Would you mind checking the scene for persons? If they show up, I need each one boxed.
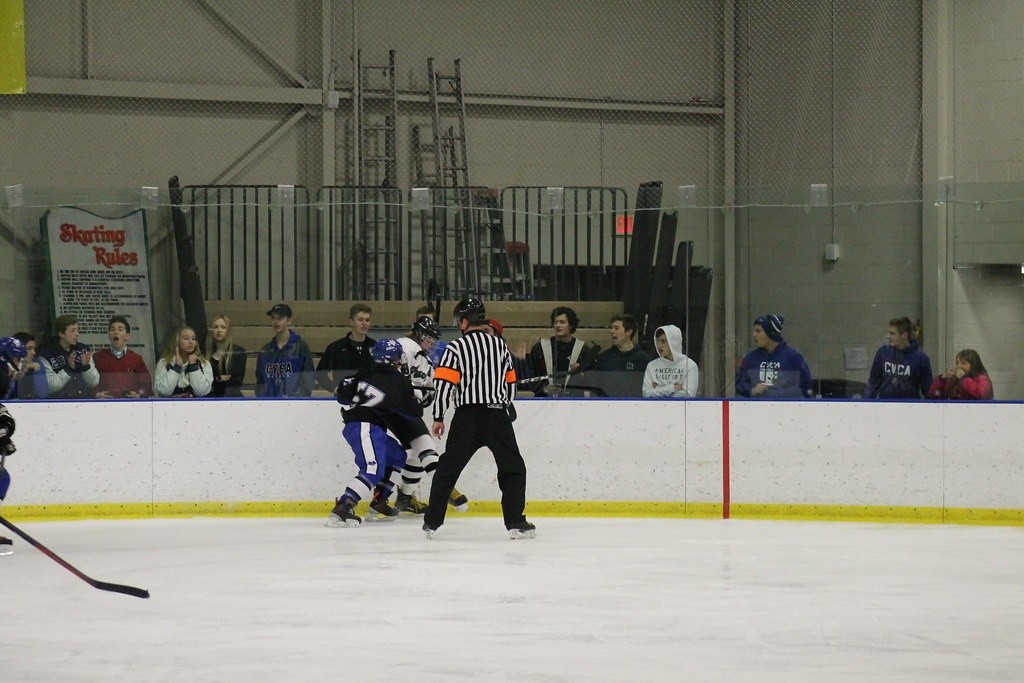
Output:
[204,311,247,398]
[483,319,534,391]
[255,303,315,398]
[734,315,815,399]
[865,314,934,400]
[640,325,699,398]
[321,316,468,528]
[416,307,447,372]
[316,303,377,397]
[0,337,28,558]
[153,324,213,397]
[928,349,995,400]
[525,303,595,398]
[87,317,151,399]
[596,314,651,373]
[422,297,536,540]
[8,332,48,399]
[38,313,101,399]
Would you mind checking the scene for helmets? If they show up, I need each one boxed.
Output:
[410,316,441,355]
[374,339,403,364]
[454,298,486,324]
[0,336,27,364]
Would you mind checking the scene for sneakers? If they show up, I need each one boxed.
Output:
[424,521,442,539]
[448,488,468,511]
[0,536,13,555]
[324,497,362,527]
[365,488,399,521]
[395,485,428,516]
[508,522,536,538]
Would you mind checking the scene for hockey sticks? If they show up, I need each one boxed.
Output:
[0,515,151,599]
[516,345,601,385]
[435,292,441,323]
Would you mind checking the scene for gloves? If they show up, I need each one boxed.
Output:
[0,429,15,455]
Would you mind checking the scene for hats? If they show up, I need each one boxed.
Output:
[488,319,503,335]
[759,315,784,340]
[267,304,292,318]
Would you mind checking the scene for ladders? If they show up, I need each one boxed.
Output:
[412,124,535,300]
[341,48,402,301]
[427,56,478,300]
